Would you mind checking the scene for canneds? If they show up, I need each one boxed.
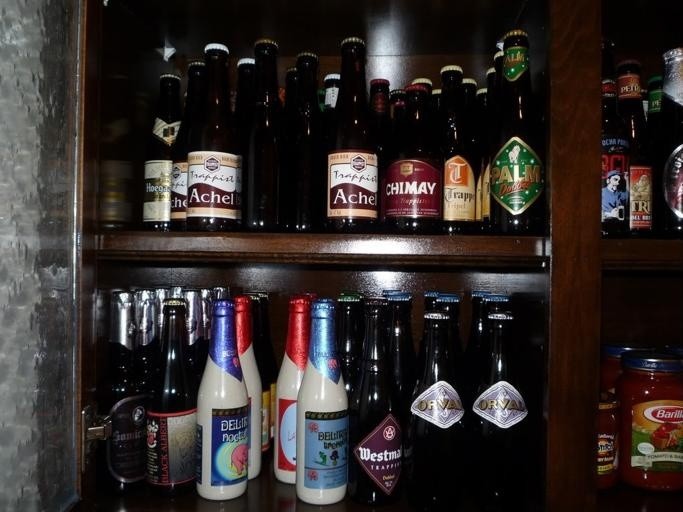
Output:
[596,341,683,500]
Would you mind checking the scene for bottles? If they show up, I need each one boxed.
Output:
[98,278,530,511]
[85,288,105,373]
[601,45,681,236]
[138,29,546,233]
[99,67,140,239]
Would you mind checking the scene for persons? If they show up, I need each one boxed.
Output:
[601,169,633,226]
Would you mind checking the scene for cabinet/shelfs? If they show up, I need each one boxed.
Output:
[577,0,682,512]
[72,0,577,511]
